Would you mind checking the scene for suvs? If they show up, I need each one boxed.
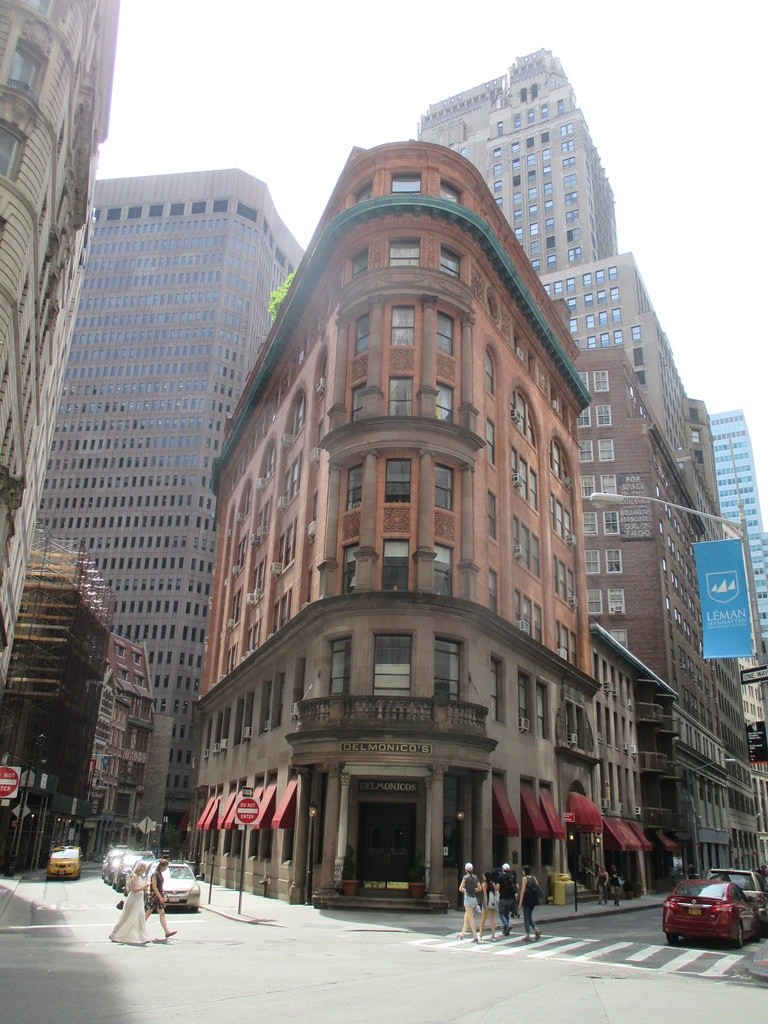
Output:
[706,868,768,938]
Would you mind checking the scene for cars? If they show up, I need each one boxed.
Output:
[662,879,762,949]
[144,862,203,913]
[101,844,198,897]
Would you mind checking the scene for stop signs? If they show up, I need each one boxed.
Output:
[0,767,19,798]
[235,797,260,823]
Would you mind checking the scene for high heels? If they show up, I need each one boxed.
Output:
[457,934,464,940]
[471,937,477,943]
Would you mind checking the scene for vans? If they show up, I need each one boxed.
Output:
[47,845,84,881]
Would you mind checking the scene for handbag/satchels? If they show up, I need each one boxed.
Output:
[161,892,169,902]
[487,890,497,909]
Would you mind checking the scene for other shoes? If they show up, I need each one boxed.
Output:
[598,900,619,906]
[522,937,532,942]
[535,929,541,940]
[511,913,520,918]
[504,925,512,936]
[165,931,177,938]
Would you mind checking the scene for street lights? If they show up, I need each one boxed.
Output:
[590,491,768,742]
[689,758,737,879]
[5,744,50,878]
[79,768,100,845]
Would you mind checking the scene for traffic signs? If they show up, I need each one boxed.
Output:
[242,786,254,798]
[740,663,768,685]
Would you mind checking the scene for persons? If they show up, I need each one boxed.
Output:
[145,859,178,939]
[494,863,519,936]
[518,865,545,941]
[594,865,608,905]
[611,864,626,906]
[108,862,151,944]
[761,862,767,880]
[457,862,483,943]
[476,871,500,941]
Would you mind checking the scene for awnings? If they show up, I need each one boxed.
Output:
[653,833,678,851]
[568,791,603,832]
[519,781,564,840]
[177,808,189,832]
[270,772,298,830]
[604,818,652,852]
[491,775,518,837]
[251,778,277,830]
[216,784,246,829]
[197,790,224,831]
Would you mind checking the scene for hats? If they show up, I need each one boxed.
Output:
[465,863,473,871]
[502,863,510,871]
